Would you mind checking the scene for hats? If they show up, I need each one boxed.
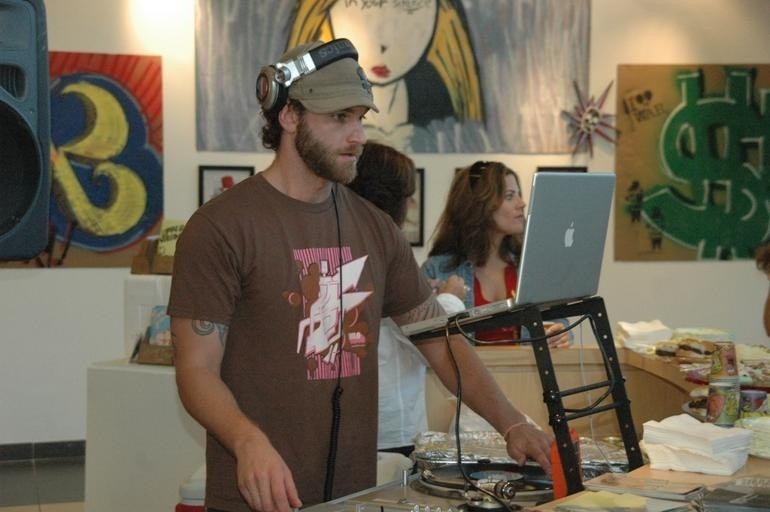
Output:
[277,41,379,115]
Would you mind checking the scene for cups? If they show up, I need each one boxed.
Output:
[741,390,767,411]
[709,341,740,396]
[705,382,739,426]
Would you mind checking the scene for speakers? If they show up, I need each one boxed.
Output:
[0,0,51,262]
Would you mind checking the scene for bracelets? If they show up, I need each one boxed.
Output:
[503,420,538,442]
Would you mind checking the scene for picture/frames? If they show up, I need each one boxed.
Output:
[195,163,257,206]
[404,168,427,248]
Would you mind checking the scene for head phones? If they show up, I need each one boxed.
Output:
[255,38,358,113]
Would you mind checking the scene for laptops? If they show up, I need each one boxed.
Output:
[401,172,616,337]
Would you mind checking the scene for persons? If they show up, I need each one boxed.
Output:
[161,36,557,511]
[333,138,432,468]
[417,160,577,355]
[271,1,489,135]
